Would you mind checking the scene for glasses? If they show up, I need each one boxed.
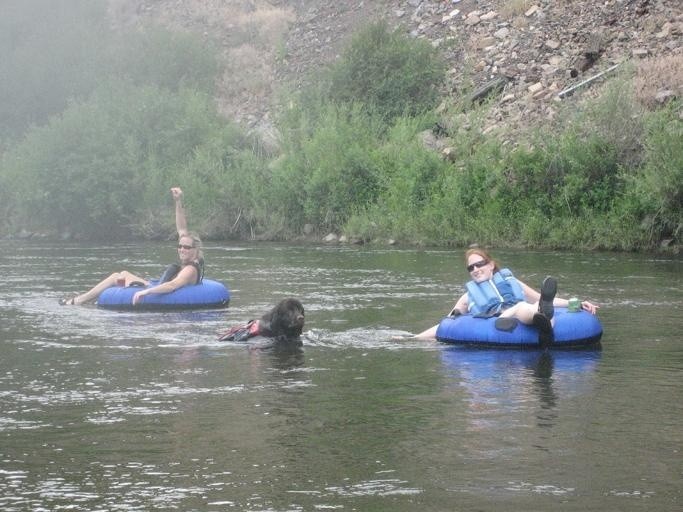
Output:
[466,259,490,273]
[177,243,197,251]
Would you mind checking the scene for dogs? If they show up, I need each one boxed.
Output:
[216,298,306,342]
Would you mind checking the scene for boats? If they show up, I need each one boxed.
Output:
[435,305,604,351]
[97,277,232,311]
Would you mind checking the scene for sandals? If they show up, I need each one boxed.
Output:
[58,298,76,306]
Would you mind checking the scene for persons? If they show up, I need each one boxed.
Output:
[418,248,600,346]
[59,187,205,306]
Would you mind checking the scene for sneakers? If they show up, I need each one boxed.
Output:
[537,276,557,320]
[532,313,555,347]
